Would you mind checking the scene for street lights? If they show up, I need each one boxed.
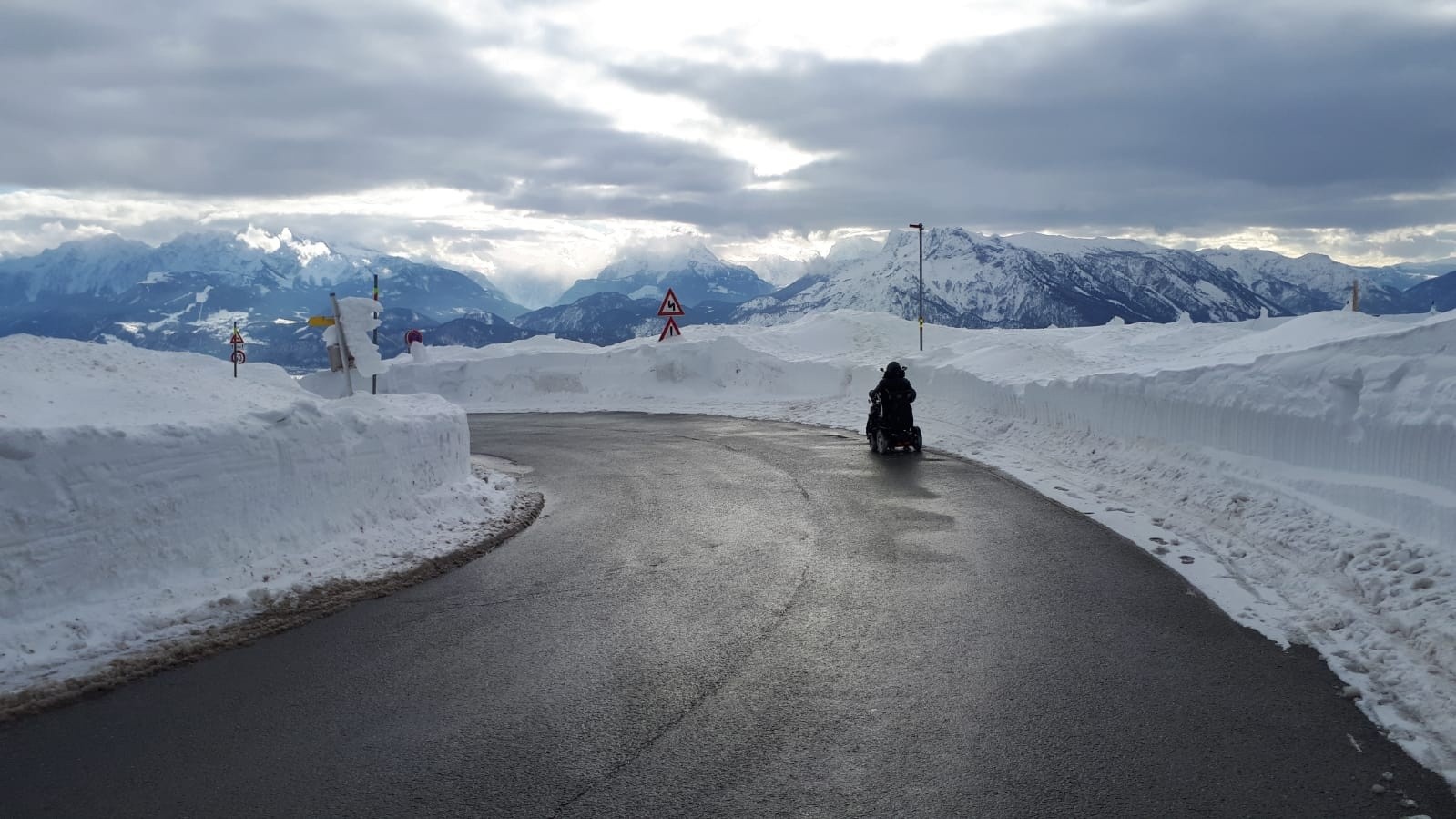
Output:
[910,223,926,350]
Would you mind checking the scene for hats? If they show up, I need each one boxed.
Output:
[887,362,900,372]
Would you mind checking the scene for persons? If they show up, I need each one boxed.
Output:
[869,363,915,432]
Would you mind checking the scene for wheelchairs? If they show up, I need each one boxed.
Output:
[865,389,924,454]
